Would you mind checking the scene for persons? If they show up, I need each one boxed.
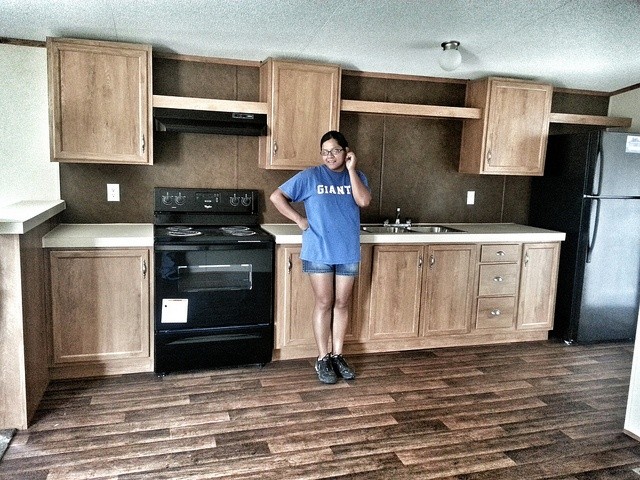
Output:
[269,130,373,383]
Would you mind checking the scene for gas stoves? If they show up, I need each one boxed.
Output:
[155,224,276,244]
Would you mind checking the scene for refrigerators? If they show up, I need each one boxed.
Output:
[527,132,639,344]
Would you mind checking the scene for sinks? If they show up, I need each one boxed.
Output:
[408,223,467,232]
[363,224,411,234]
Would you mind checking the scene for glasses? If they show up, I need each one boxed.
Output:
[320,148,345,155]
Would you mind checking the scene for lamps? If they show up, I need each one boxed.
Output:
[438,41,462,71]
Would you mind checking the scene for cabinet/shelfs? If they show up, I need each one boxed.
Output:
[278,245,362,346]
[457,75,554,177]
[42,246,154,382]
[515,242,560,330]
[257,56,343,171]
[367,243,477,341]
[472,243,521,331]
[45,35,156,166]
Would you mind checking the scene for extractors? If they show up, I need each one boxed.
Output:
[155,108,268,137]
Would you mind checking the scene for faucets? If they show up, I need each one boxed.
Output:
[396,207,403,225]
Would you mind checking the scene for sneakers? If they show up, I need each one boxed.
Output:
[332,354,356,379]
[315,353,337,383]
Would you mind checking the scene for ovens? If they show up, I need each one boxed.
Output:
[152,245,273,377]
